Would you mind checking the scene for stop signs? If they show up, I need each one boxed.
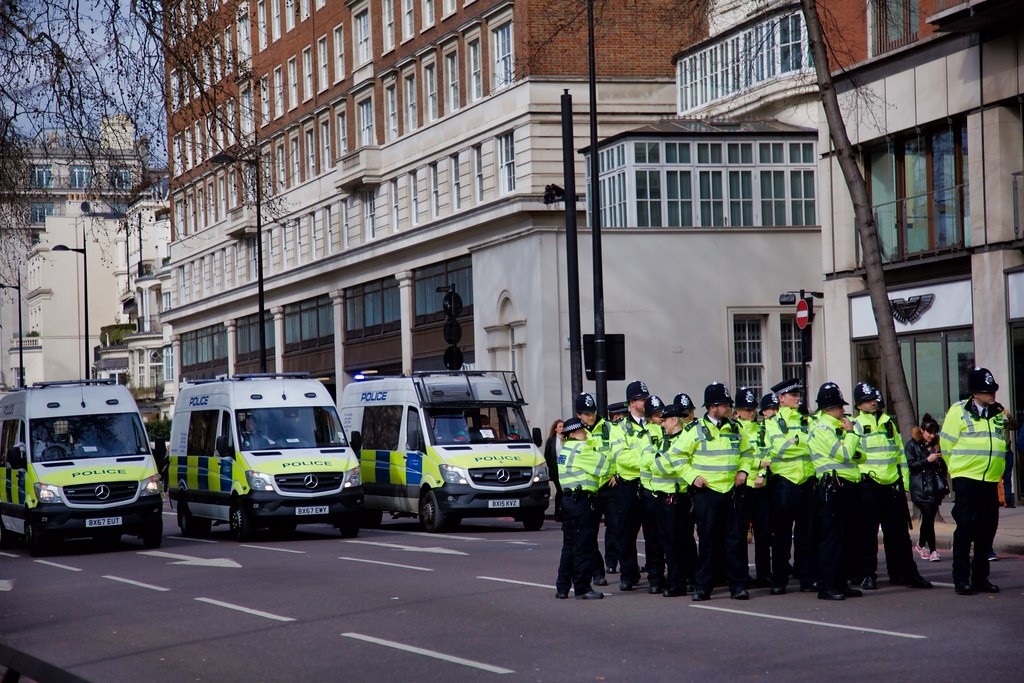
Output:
[796,299,809,329]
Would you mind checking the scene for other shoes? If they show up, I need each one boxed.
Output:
[554,514,560,522]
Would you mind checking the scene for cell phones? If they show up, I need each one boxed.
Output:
[936,451,941,455]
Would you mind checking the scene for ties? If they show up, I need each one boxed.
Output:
[982,409,986,417]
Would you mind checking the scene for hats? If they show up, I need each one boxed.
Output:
[561,418,588,436]
[771,378,804,398]
[606,402,629,414]
[660,405,685,419]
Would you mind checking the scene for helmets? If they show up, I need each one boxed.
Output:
[759,393,780,416]
[627,381,650,402]
[734,388,758,410]
[575,392,597,413]
[701,382,734,408]
[816,382,850,412]
[644,395,665,417]
[854,384,877,410]
[967,368,999,393]
[674,393,696,414]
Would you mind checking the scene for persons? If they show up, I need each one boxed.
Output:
[241,415,275,444]
[939,368,1006,594]
[1003,409,1018,508]
[555,378,932,600]
[473,417,498,438]
[905,412,946,560]
[544,419,565,521]
[162,455,169,495]
[13,425,48,462]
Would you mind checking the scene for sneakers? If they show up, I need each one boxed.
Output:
[930,551,940,562]
[915,541,929,559]
[988,551,998,560]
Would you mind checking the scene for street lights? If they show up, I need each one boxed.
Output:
[52,224,90,386]
[211,126,267,373]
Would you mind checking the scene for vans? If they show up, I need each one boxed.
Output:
[338,369,551,532]
[168,372,365,538]
[0,380,164,556]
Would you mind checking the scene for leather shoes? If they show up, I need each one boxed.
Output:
[555,566,999,601]
